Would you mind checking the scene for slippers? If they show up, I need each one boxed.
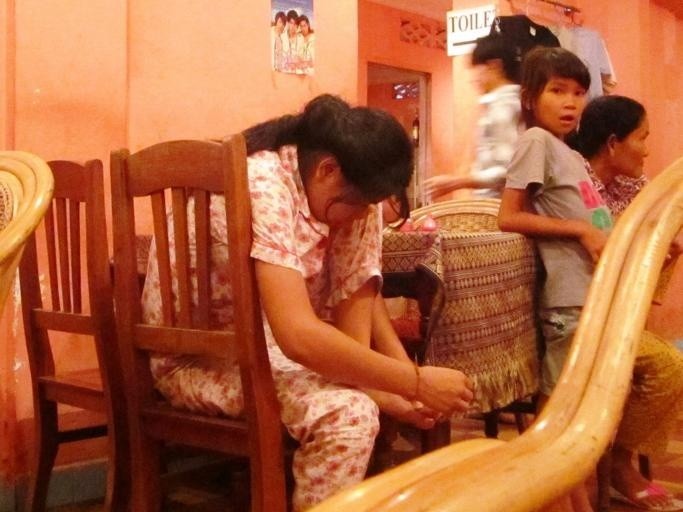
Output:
[610,483,682,511]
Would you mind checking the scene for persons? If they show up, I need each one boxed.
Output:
[422,33,523,201]
[565,94,683,510]
[272,9,314,74]
[497,46,616,512]
[401,366,419,401]
[140,94,475,512]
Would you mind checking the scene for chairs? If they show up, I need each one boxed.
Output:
[418,175,538,437]
[110,135,396,512]
[17,158,123,512]
[1,148,55,512]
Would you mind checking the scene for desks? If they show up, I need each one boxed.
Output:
[110,230,543,455]
